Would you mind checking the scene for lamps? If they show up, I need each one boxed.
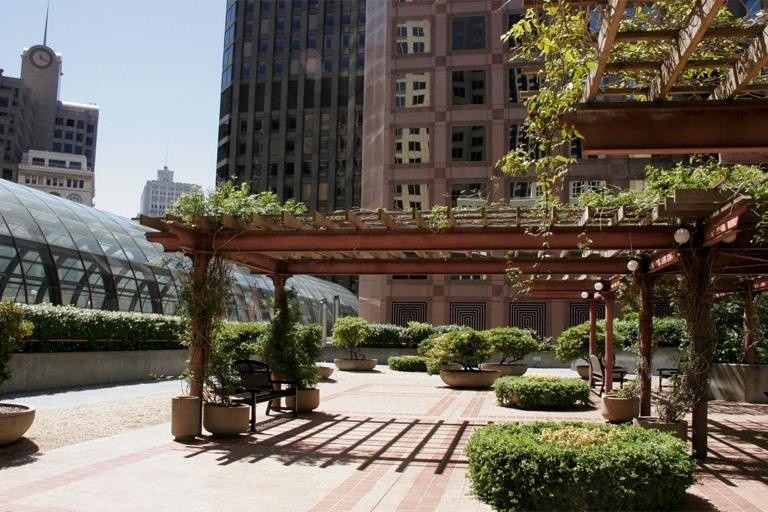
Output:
[624,256,641,273]
[579,290,590,299]
[592,280,604,292]
[672,221,691,247]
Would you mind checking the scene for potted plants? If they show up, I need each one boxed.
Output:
[0,296,40,446]
[415,331,464,373]
[427,330,503,390]
[277,322,321,415]
[329,314,379,372]
[488,372,596,411]
[479,323,554,378]
[295,320,332,380]
[182,320,256,437]
[601,371,645,422]
[554,315,630,379]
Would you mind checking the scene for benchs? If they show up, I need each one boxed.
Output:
[654,357,686,399]
[586,351,630,397]
[228,358,300,433]
